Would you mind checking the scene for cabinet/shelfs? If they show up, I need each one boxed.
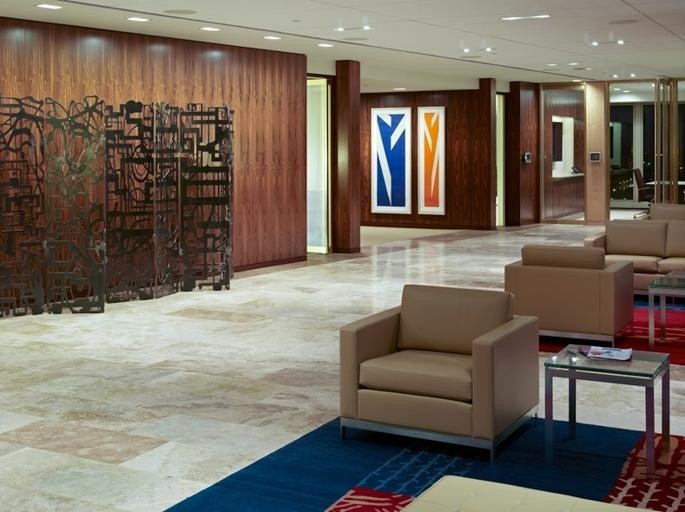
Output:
[552,180,585,218]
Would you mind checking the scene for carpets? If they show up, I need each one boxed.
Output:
[162,415,684,510]
[539,296,685,365]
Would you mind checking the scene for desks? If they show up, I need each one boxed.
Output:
[644,180,685,201]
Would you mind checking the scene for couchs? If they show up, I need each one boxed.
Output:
[584,203,685,298]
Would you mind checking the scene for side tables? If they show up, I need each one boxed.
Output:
[536,341,672,480]
[645,270,685,345]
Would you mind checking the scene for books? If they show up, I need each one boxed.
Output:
[568,349,587,360]
[587,345,633,362]
[579,346,615,361]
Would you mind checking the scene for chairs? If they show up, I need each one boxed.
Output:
[633,169,656,219]
[505,243,635,351]
[334,282,540,463]
